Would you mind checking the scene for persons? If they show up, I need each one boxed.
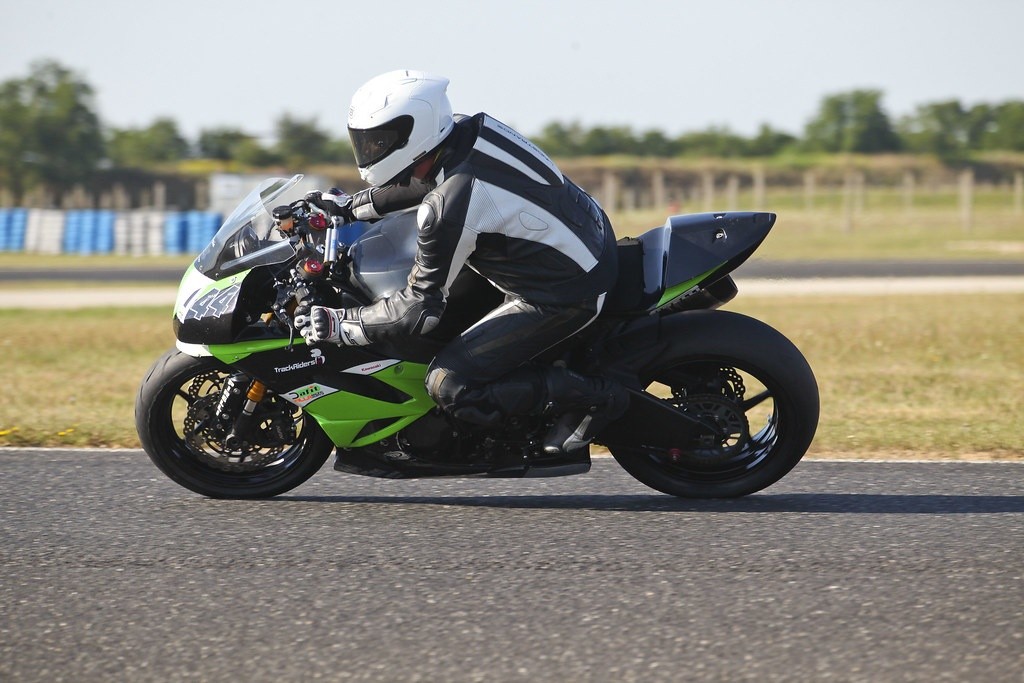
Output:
[295,65,630,452]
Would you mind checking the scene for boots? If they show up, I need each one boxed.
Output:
[542,360,630,453]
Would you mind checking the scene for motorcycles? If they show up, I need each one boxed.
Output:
[135,174,820,501]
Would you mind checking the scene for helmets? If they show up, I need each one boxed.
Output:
[347,68,455,188]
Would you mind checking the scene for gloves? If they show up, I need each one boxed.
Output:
[293,306,373,349]
[321,186,384,227]
